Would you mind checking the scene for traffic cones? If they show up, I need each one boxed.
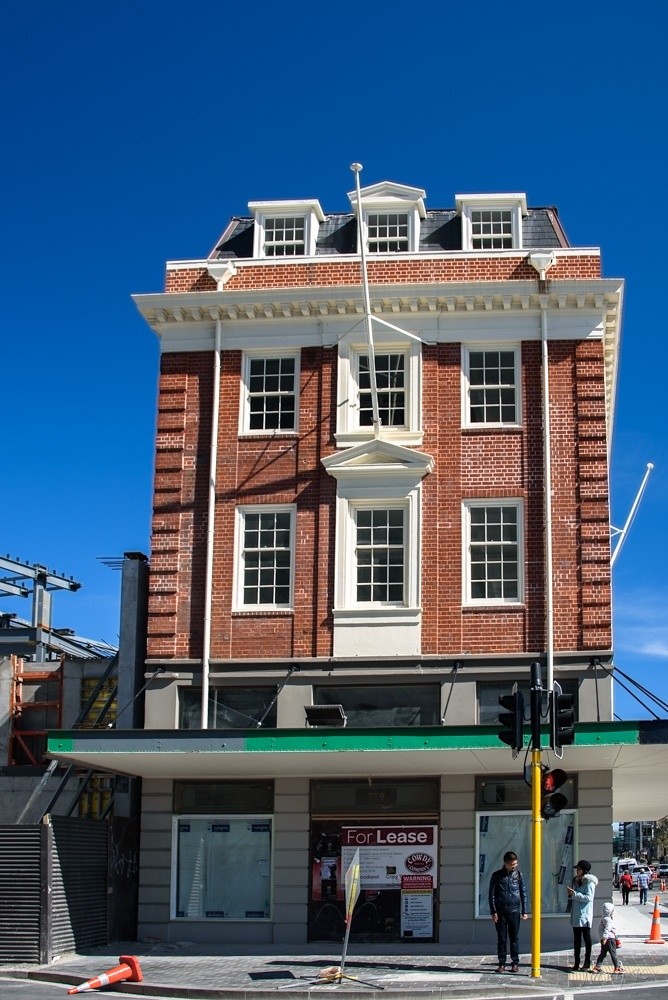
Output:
[645,896,665,944]
[653,871,657,880]
[68,954,143,995]
[663,881,666,891]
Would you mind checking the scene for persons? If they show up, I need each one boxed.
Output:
[593,902,626,973]
[619,870,632,905]
[636,868,649,905]
[566,860,599,972]
[489,851,528,972]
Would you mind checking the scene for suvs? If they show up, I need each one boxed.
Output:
[614,858,668,888]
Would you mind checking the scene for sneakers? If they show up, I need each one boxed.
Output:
[580,967,590,972]
[613,968,626,973]
[566,968,578,973]
[593,967,605,974]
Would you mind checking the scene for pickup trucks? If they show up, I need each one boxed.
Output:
[630,865,653,890]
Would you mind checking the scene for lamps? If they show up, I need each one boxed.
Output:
[304,705,347,730]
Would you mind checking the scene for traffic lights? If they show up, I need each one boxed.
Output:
[553,680,574,759]
[497,692,523,754]
[541,768,568,820]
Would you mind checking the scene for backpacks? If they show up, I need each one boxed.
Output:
[622,876,630,890]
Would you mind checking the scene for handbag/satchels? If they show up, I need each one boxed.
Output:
[616,940,621,948]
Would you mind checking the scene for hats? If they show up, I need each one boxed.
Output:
[573,860,591,874]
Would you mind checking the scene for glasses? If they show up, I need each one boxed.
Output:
[578,867,582,870]
[505,861,518,866]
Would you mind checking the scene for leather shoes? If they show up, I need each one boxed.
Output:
[496,962,505,972]
[512,963,519,972]
